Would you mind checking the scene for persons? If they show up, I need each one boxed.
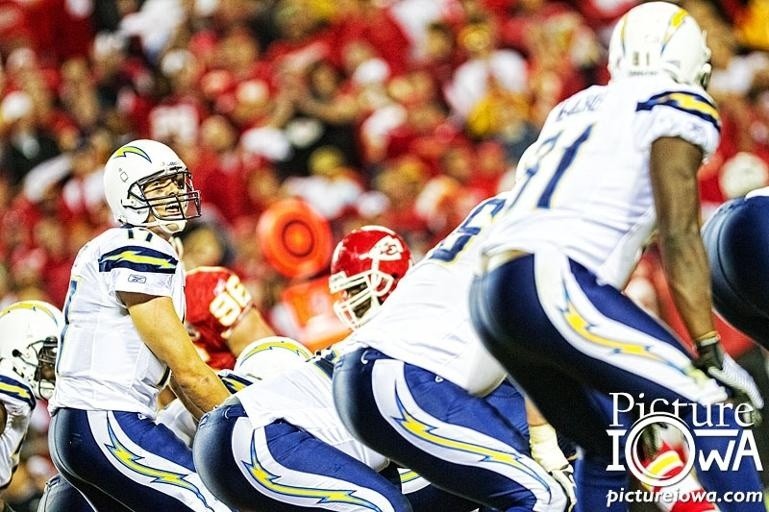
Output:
[1,1,769,511]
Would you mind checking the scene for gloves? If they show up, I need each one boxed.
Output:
[692,328,764,410]
[528,423,578,511]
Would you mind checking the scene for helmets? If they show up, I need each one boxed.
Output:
[0,300,65,402]
[607,0,712,92]
[330,223,417,332]
[102,138,201,233]
[234,335,315,381]
